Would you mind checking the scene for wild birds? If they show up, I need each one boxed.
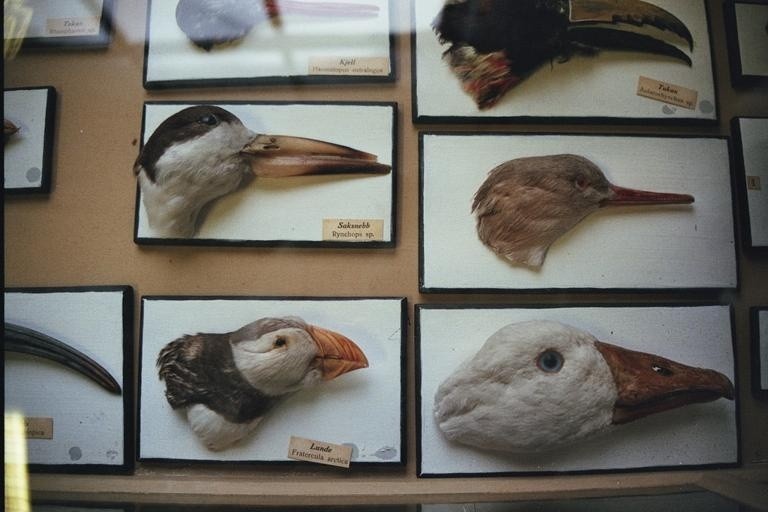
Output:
[433,319,736,455]
[176,0,380,52]
[132,105,393,238]
[471,154,695,268]
[155,317,369,453]
[431,0,694,109]
